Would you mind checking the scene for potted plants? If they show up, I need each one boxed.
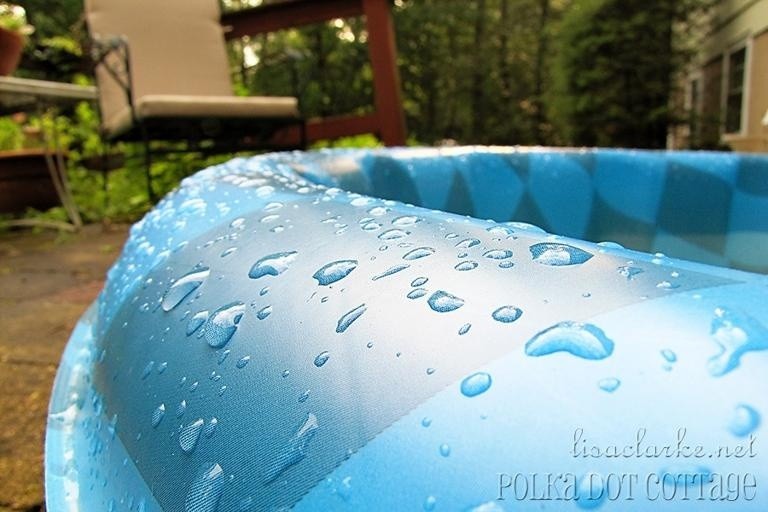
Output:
[0,2,35,77]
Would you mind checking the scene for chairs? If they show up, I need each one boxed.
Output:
[81,1,312,215]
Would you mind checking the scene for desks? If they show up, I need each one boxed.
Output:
[0,73,102,236]
